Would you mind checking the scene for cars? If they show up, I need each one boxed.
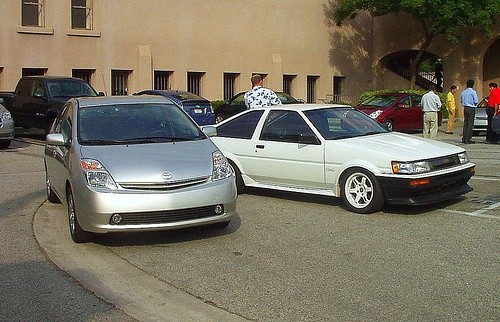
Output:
[213,91,304,124]
[42,95,237,244]
[339,93,442,133]
[133,90,216,127]
[474,96,488,135]
[199,103,476,214]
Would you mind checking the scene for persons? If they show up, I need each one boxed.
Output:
[420,86,441,140]
[480,83,500,144]
[445,85,457,134]
[461,80,479,144]
[243,74,282,109]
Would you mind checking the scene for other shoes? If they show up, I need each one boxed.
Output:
[446,132,453,134]
[485,140,489,144]
[462,139,476,143]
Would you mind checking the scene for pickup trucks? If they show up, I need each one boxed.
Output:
[0,75,106,139]
